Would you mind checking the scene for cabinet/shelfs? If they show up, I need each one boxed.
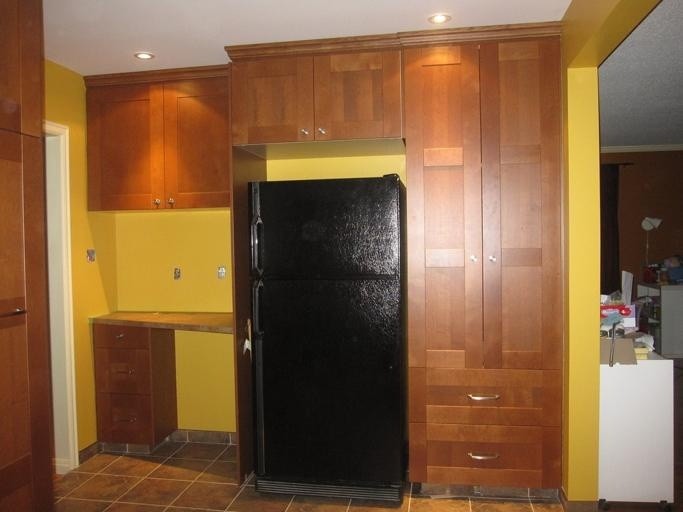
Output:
[404,424,561,487]
[230,50,405,143]
[91,328,151,450]
[83,79,235,211]
[635,283,683,359]
[407,368,561,424]
[403,38,562,370]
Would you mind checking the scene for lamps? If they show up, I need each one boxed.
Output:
[641,215,664,264]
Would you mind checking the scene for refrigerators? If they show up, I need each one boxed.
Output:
[247,177,407,501]
